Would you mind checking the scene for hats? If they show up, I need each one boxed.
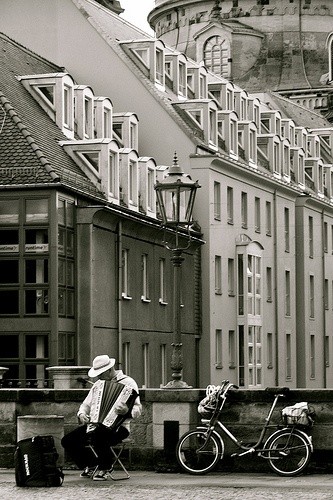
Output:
[88,355,115,378]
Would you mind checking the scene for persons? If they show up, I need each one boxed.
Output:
[62,355,142,481]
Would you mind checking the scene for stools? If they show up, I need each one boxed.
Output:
[84,444,130,481]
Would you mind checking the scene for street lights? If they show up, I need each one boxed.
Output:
[153,151,202,389]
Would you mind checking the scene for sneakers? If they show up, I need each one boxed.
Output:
[79,467,114,480]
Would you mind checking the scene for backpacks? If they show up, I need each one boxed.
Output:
[12,433,64,488]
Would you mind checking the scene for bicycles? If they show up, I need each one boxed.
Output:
[175,378,315,477]
